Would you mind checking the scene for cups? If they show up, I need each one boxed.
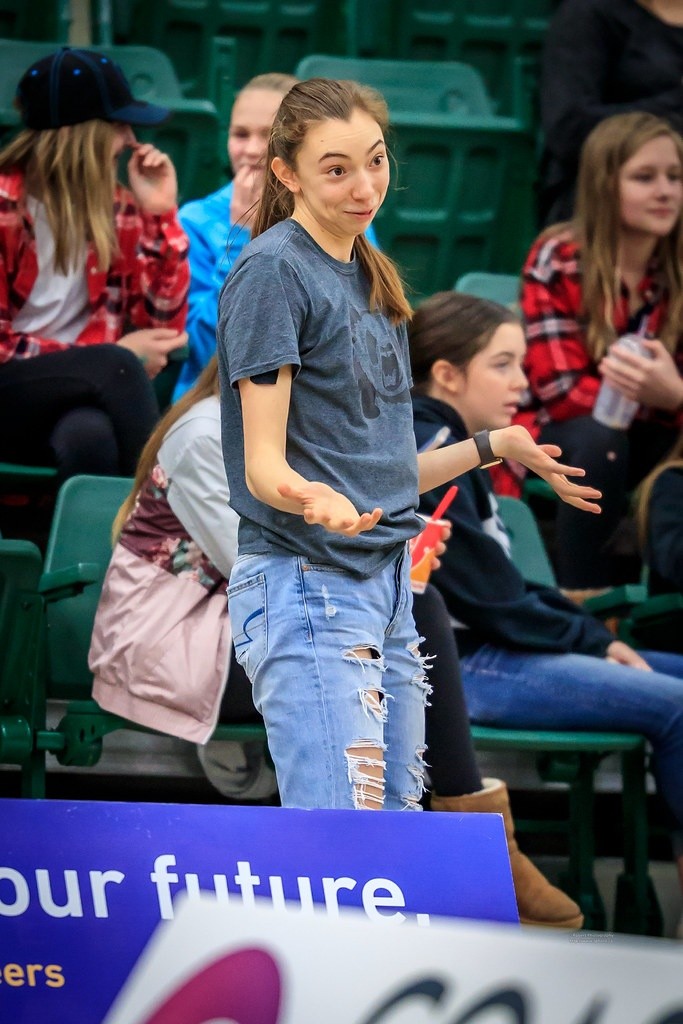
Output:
[407,512,451,595]
[592,333,658,431]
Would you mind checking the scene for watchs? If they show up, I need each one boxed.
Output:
[473,429,504,470]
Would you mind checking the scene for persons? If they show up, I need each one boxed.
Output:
[0,46,190,488]
[407,293,683,887]
[88,353,587,932]
[503,110,683,587]
[217,80,606,813]
[171,71,381,404]
[629,434,683,654]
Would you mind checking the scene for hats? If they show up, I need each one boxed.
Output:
[17,45,168,125]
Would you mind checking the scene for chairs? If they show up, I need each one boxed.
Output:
[0,0,683,938]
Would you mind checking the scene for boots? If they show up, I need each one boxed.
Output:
[432,775,585,934]
